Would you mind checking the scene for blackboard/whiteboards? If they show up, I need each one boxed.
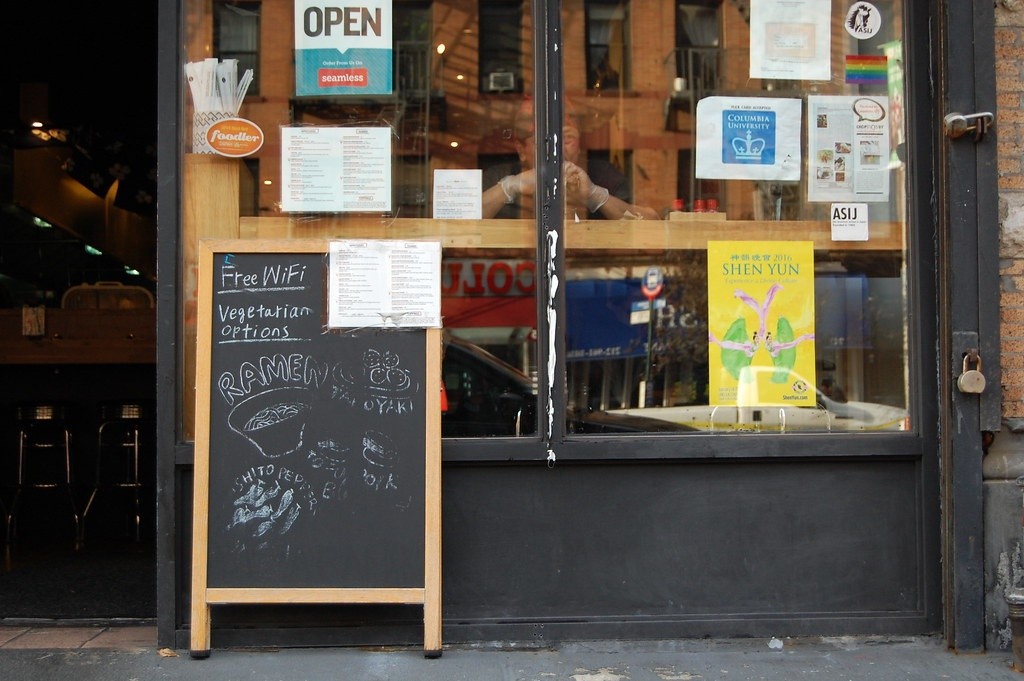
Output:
[196,239,443,605]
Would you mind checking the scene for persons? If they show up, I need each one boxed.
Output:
[592,57,621,120]
[467,57,525,219]
[482,90,660,220]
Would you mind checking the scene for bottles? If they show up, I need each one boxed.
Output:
[22,261,46,339]
